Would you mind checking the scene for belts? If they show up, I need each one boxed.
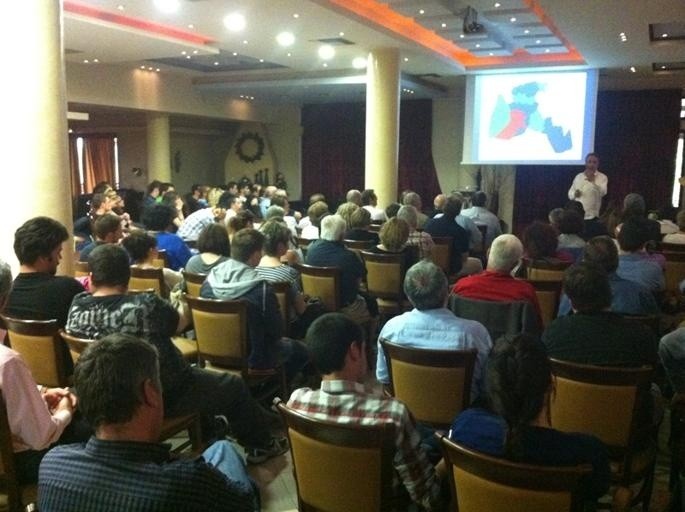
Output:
[339,297,354,309]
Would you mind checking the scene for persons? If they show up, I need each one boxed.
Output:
[0,344,89,485]
[567,152,609,221]
[73,176,431,387]
[35,333,259,512]
[375,191,684,445]
[450,335,618,512]
[288,316,452,512]
[0,216,88,384]
[66,244,289,464]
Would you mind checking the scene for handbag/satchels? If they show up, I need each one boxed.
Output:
[303,296,326,324]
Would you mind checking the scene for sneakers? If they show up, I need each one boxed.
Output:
[246,435,288,464]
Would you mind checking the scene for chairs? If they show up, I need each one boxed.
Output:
[0,222,684,510]
